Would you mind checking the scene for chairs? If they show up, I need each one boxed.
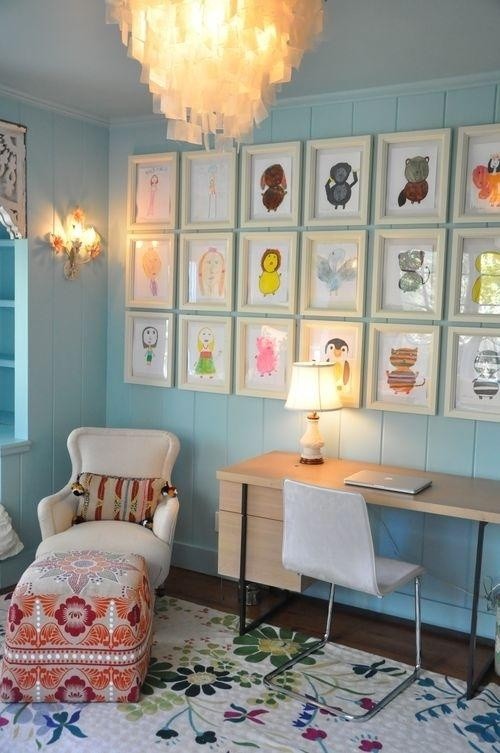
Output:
[264,480,423,723]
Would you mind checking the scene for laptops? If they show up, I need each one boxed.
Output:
[345,469,433,495]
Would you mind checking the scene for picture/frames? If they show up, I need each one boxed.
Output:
[236,317,296,400]
[444,326,500,422]
[299,319,365,408]
[366,323,442,415]
[448,227,500,323]
[124,311,175,387]
[452,123,500,222]
[238,231,298,314]
[126,233,177,309]
[127,152,178,230]
[179,232,235,311]
[241,141,302,227]
[300,230,368,317]
[375,128,451,224]
[371,228,446,320]
[181,148,238,230]
[178,314,234,395]
[305,135,373,226]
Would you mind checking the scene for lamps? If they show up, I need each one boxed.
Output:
[107,1,324,152]
[47,207,103,280]
[283,361,344,464]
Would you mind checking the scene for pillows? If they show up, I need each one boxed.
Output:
[72,472,176,528]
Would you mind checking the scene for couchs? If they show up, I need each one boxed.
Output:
[36,427,180,596]
[3,551,154,702]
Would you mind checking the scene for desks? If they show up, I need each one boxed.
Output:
[218,449,499,699]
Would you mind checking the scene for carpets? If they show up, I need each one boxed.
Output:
[0,585,499,753]
